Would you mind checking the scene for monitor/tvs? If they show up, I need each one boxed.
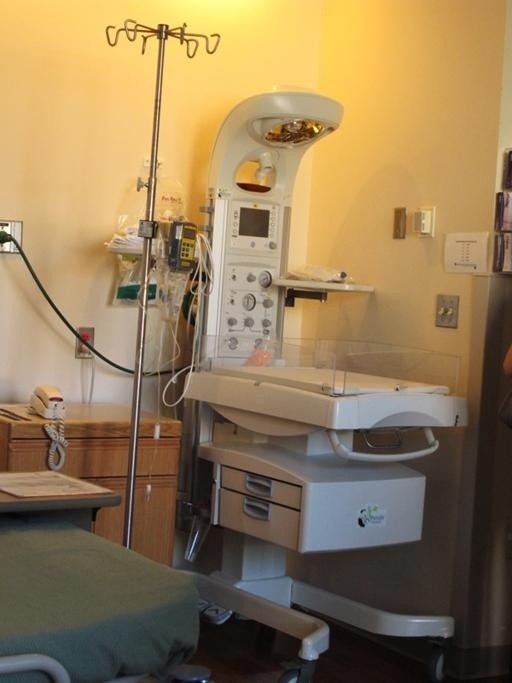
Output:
[239,207,270,238]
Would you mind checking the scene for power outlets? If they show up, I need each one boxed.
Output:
[1,219,22,254]
[76,326,95,360]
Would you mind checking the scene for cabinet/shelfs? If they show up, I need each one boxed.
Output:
[195,441,426,554]
[0,403,182,569]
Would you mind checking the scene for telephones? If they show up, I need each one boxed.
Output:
[29,383,66,420]
